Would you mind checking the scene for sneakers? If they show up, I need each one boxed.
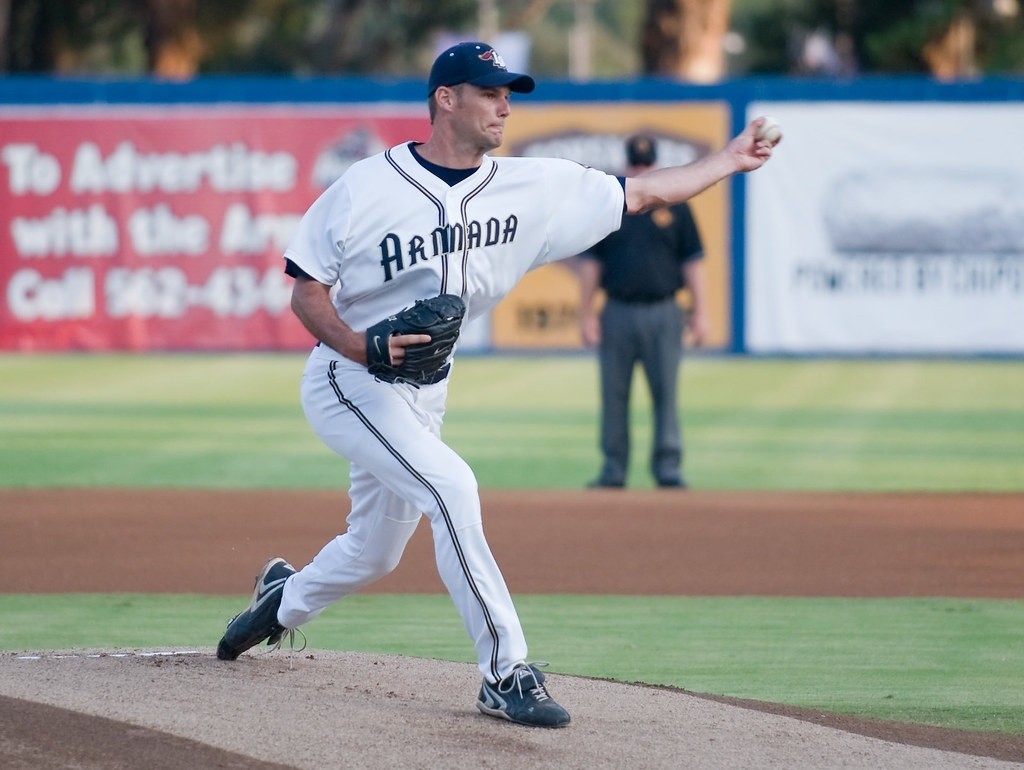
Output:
[476,660,572,729]
[216,554,308,663]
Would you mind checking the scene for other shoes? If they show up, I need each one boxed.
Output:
[586,482,625,488]
[654,478,684,488]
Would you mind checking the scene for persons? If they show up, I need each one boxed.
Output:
[575,135,708,490]
[217,41,783,726]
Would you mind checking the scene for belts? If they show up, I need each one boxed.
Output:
[607,292,677,303]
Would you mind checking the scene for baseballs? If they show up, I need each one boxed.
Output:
[756,115,781,145]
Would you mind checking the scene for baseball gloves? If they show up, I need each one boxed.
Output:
[367,294,467,386]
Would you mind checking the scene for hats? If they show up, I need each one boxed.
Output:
[426,41,536,96]
[625,135,658,161]
